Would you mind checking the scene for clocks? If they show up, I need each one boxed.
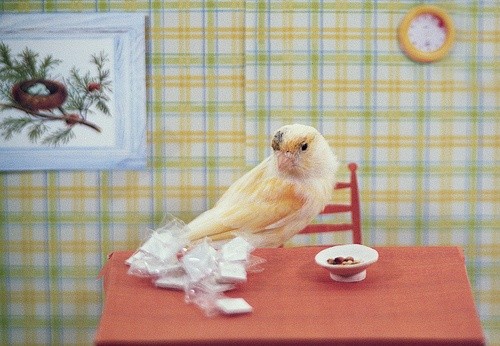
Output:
[399,5,456,63]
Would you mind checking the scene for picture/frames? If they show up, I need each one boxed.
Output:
[0,12,148,171]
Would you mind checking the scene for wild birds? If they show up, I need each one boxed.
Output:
[182,123,338,249]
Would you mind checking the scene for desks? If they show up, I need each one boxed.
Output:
[92,244,487,346]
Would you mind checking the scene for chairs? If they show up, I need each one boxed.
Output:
[278,163,362,248]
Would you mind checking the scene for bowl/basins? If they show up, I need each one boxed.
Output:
[315,244,379,282]
